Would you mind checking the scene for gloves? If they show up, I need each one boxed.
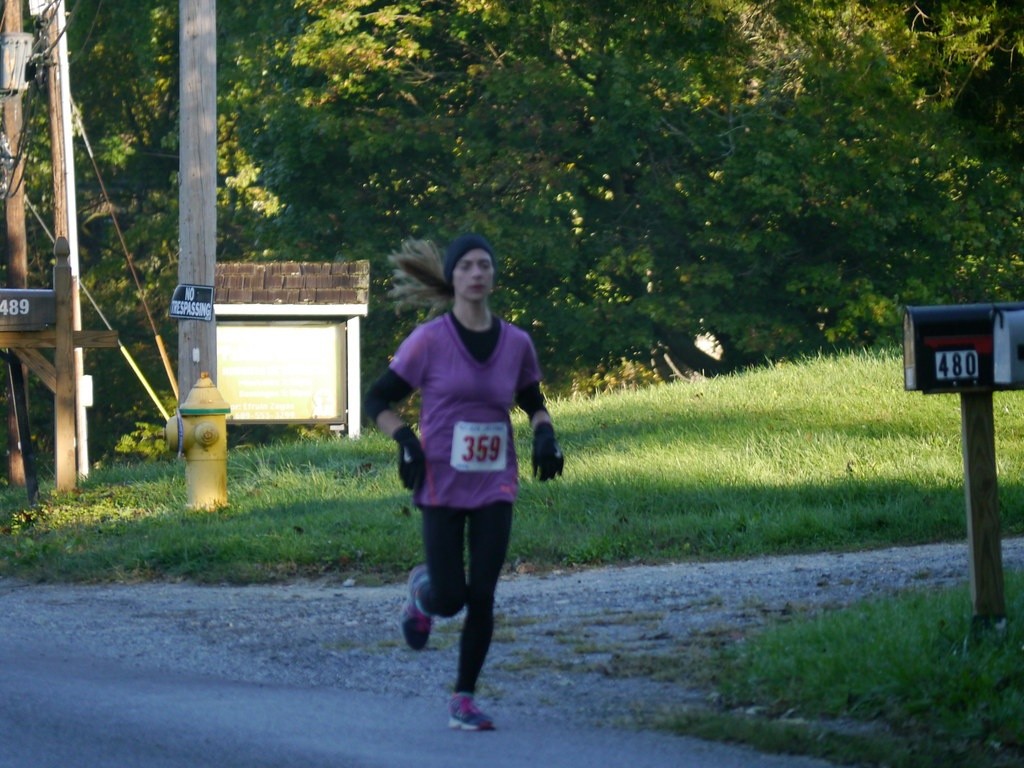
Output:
[394,424,424,490]
[533,423,564,481]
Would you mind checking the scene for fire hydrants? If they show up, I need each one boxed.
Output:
[152,371,231,513]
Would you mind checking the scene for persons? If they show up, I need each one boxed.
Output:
[362,231,566,731]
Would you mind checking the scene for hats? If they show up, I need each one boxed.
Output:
[444,235,496,284]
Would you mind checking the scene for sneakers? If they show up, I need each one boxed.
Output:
[449,692,492,730]
[400,565,434,650]
